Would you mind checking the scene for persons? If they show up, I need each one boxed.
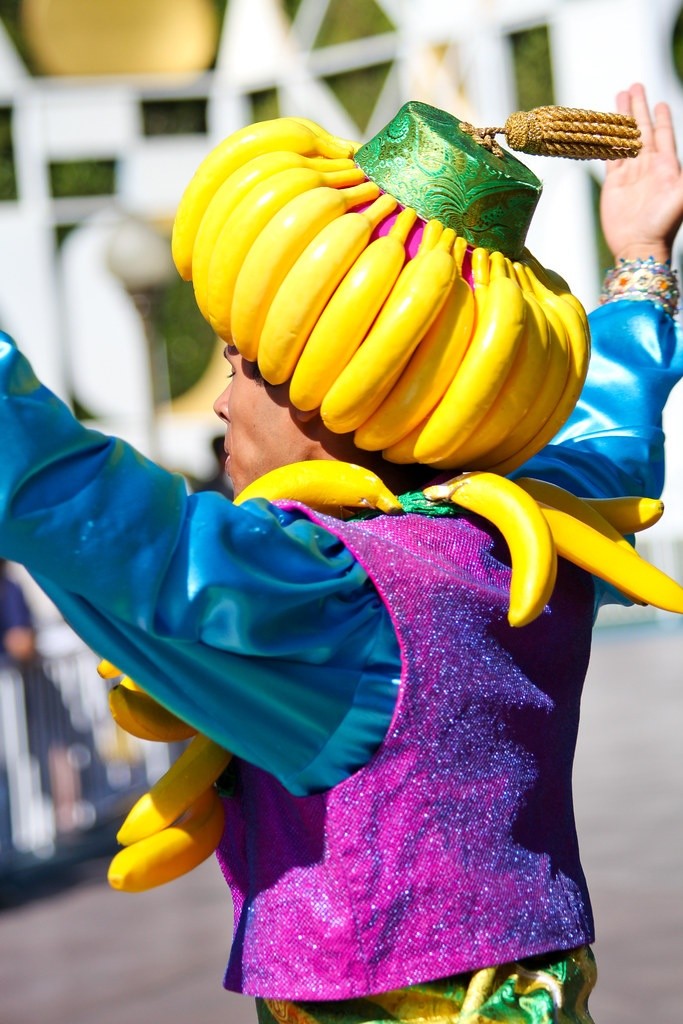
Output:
[0,576,111,844]
[0,83,683,1024]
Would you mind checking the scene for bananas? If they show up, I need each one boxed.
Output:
[170,118,593,477]
[94,457,683,894]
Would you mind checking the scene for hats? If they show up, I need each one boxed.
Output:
[168,97,638,473]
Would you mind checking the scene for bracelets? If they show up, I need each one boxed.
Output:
[599,256,679,317]
